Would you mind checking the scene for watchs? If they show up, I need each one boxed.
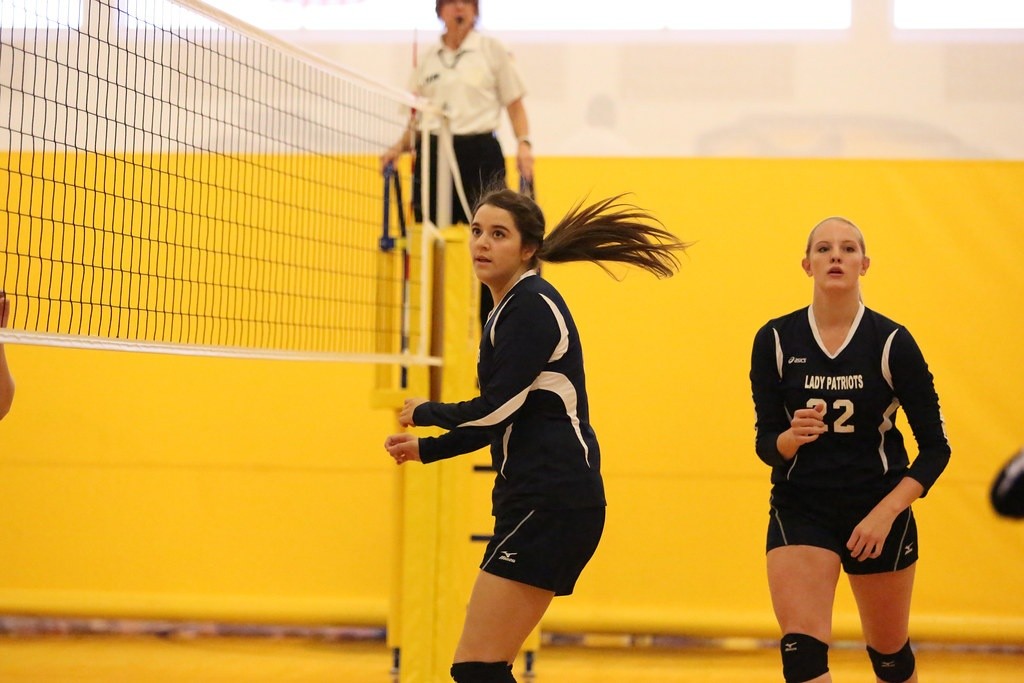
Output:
[515,134,532,147]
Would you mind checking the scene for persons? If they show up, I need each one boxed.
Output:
[382,0,534,395]
[748,218,953,683]
[989,447,1024,522]
[0,290,17,421]
[384,169,701,683]
